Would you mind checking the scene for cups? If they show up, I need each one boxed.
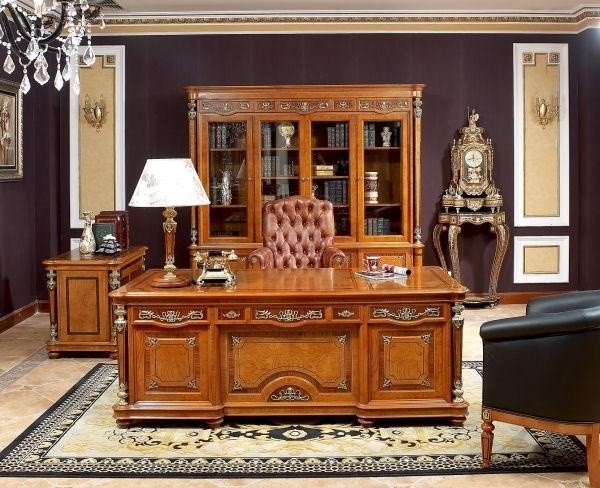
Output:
[361,255,381,273]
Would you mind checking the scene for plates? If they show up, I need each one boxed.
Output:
[360,269,387,276]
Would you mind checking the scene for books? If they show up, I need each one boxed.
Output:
[354,262,411,280]
[93,210,129,250]
[210,122,391,236]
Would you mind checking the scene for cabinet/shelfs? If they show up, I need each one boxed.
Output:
[42,244,149,360]
[188,242,425,267]
[254,84,357,244]
[357,83,427,243]
[181,84,254,268]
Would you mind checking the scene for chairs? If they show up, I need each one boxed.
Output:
[248,194,348,269]
[479,288,599,488]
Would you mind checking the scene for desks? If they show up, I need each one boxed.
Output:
[108,266,470,429]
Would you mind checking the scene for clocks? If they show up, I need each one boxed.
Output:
[433,104,510,309]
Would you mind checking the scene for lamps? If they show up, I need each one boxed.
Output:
[81,93,106,133]
[0,0,105,94]
[534,91,561,130]
[128,158,213,287]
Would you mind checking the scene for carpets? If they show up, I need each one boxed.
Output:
[1,362,588,478]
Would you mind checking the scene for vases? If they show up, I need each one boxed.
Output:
[220,170,232,205]
[78,210,96,259]
[379,125,393,147]
[364,171,379,204]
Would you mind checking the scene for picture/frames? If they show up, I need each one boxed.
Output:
[0,78,24,181]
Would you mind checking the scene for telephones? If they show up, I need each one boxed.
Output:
[193,249,240,287]
